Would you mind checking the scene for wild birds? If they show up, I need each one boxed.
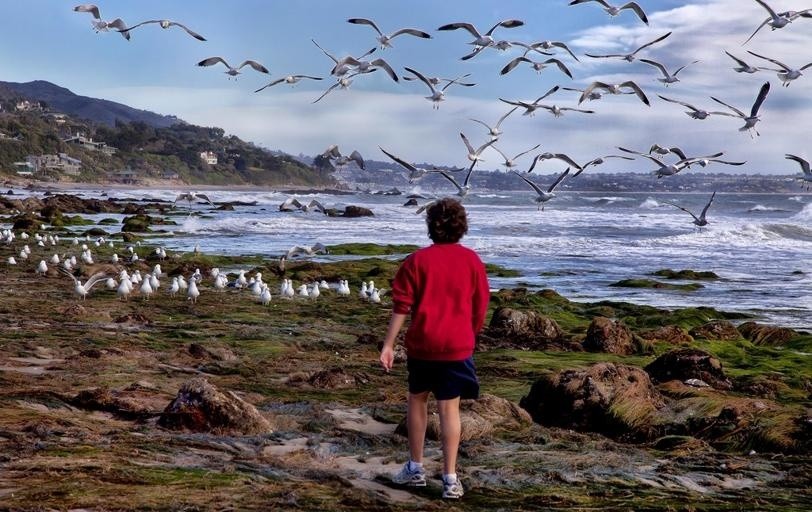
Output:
[72,4,130,41]
[0,224,387,303]
[378,146,466,184]
[512,168,570,211]
[662,190,715,228]
[572,153,635,179]
[321,145,366,175]
[434,158,477,200]
[193,56,269,81]
[784,153,812,191]
[528,151,583,173]
[115,19,207,41]
[459,132,498,164]
[618,146,723,179]
[280,198,328,217]
[674,155,748,170]
[309,0,808,140]
[174,192,217,209]
[484,140,541,173]
[650,143,691,171]
[254,75,324,93]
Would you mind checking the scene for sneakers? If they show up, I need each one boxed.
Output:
[392,463,426,486]
[443,473,464,498]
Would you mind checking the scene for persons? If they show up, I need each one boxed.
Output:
[379,198,490,499]
